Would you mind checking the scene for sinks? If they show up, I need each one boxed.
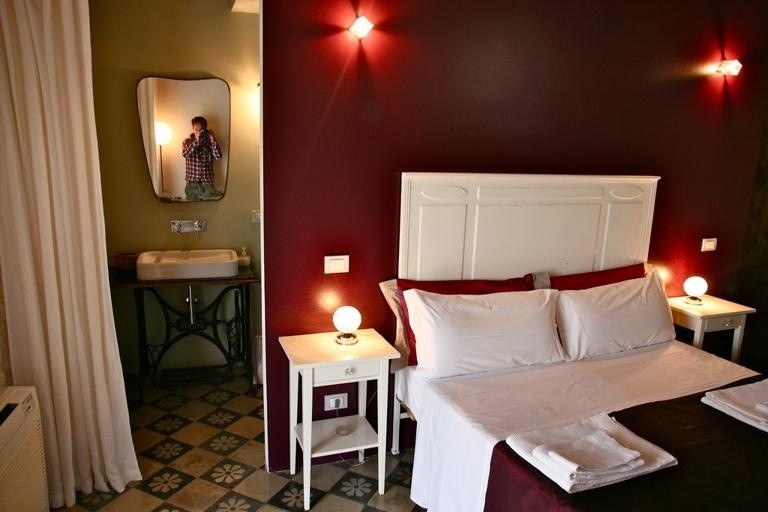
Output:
[136,249,238,279]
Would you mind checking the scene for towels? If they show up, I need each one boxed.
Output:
[695,374,767,436]
[499,410,682,499]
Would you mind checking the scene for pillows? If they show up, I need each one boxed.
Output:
[545,256,657,350]
[396,286,574,383]
[377,269,552,376]
[551,265,677,366]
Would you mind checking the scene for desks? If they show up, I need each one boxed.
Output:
[107,264,261,407]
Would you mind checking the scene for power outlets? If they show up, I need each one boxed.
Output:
[321,390,350,412]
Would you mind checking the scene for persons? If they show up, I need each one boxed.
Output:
[181,116,221,198]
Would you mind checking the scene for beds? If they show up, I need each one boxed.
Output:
[378,171,766,510]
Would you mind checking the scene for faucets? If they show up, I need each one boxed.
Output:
[193,219,207,232]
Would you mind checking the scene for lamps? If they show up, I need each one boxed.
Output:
[716,57,744,78]
[346,13,375,43]
[679,271,710,306]
[150,121,173,199]
[331,304,363,346]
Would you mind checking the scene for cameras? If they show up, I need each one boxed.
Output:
[199,129,208,137]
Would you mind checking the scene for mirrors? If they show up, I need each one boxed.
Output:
[133,72,232,205]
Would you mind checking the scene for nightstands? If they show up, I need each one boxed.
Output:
[277,324,403,511]
[665,288,760,364]
